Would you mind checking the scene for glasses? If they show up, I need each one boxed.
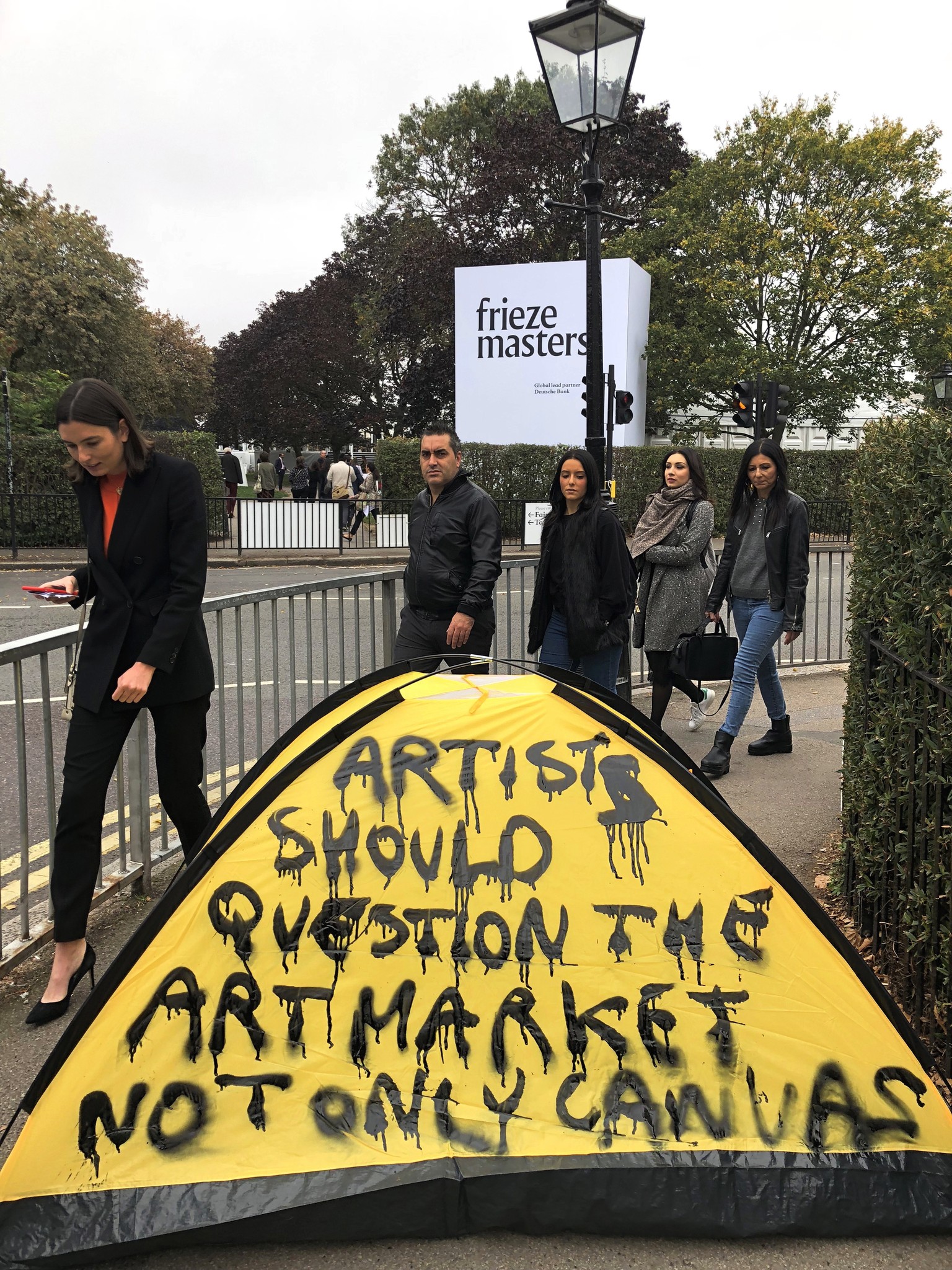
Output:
[322,453,326,454]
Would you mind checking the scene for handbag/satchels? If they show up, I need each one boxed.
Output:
[668,615,738,680]
[252,463,262,494]
[354,491,369,510]
[324,480,331,494]
[332,485,349,499]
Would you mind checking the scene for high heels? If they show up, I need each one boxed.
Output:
[26,943,97,1024]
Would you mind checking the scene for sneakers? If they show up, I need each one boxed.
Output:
[689,688,715,730]
[342,516,353,541]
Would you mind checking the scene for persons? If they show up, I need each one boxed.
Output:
[522,448,639,697]
[253,449,382,543]
[700,436,812,776]
[633,446,716,735]
[390,423,503,677]
[19,376,223,1031]
[219,447,244,518]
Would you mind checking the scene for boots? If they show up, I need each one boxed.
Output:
[700,731,735,775]
[748,714,793,756]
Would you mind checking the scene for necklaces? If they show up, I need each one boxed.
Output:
[105,476,130,495]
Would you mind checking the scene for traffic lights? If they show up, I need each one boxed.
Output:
[732,380,753,427]
[615,391,633,424]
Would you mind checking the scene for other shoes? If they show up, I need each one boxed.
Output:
[228,511,235,518]
[279,489,286,491]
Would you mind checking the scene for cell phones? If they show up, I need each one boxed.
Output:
[21,586,78,595]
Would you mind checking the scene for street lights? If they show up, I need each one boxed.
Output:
[528,0,648,703]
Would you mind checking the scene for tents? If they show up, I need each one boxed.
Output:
[0,658,952,1259]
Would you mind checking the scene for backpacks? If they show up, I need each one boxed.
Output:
[597,507,638,619]
[686,497,716,592]
